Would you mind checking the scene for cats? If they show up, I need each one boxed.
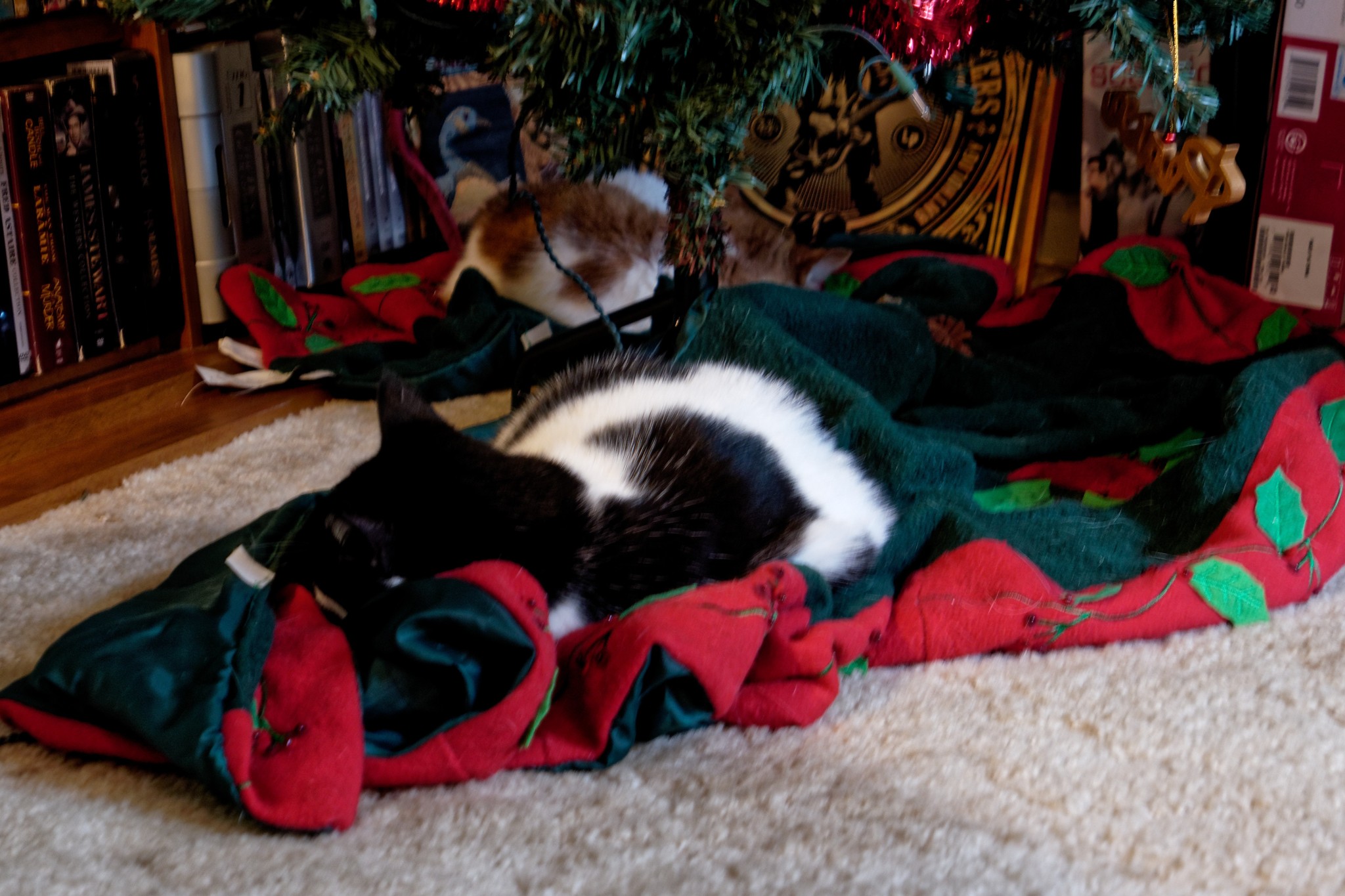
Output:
[426,163,854,338]
[253,336,908,640]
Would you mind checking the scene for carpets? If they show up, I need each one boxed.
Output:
[2,399,1341,894]
